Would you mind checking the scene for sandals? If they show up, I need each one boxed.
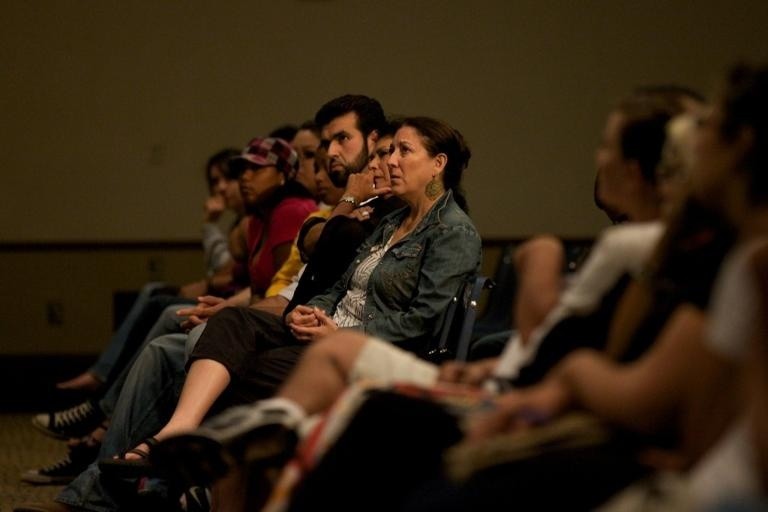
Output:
[98,436,158,478]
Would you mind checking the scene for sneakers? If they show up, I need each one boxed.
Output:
[32,401,104,440]
[57,378,97,391]
[20,455,75,484]
[142,396,305,491]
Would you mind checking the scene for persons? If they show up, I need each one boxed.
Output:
[282,118,408,321]
[283,61,768,512]
[54,95,385,512]
[595,62,767,512]
[200,144,247,278]
[151,83,706,488]
[265,146,346,298]
[97,115,482,512]
[291,121,321,196]
[231,137,317,304]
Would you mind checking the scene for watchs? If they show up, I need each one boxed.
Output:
[338,193,359,209]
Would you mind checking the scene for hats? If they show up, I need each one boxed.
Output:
[228,136,300,180]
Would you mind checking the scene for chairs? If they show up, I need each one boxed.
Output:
[438,273,496,365]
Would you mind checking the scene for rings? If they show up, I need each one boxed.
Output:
[362,211,369,216]
